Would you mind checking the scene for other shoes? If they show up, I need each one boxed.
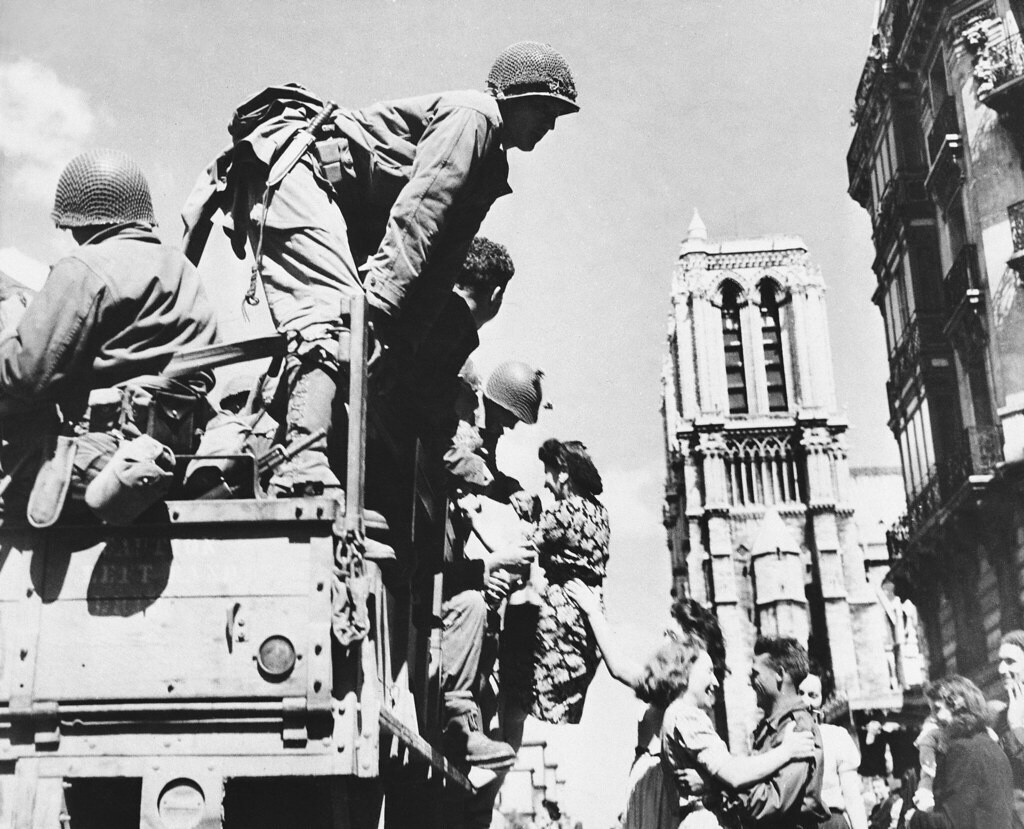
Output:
[264,465,346,511]
[440,709,516,772]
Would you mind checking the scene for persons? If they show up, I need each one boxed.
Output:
[441,494,538,770]
[333,237,516,486]
[869,629,1024,829]
[182,40,581,497]
[496,440,611,750]
[1,146,218,530]
[564,576,867,829]
[440,360,542,523]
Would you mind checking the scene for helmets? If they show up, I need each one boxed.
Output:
[51,150,159,229]
[483,361,544,424]
[484,41,581,116]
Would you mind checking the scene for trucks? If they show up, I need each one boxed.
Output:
[0,285,494,829]
